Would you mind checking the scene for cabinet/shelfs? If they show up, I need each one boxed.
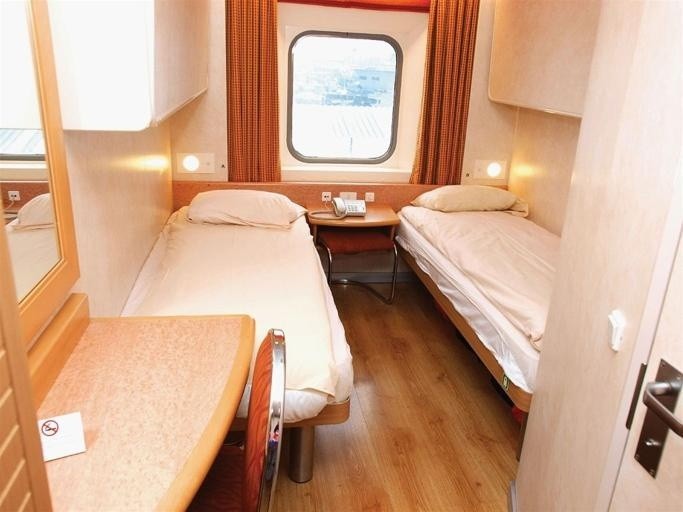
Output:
[488,1,604,121]
[47,0,210,134]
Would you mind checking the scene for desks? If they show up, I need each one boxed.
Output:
[32,316,253,512]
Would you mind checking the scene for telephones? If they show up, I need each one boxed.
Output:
[333,197,367,217]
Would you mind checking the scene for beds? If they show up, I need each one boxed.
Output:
[120,206,351,472]
[396,206,563,458]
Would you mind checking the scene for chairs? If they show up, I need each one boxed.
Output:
[184,330,288,511]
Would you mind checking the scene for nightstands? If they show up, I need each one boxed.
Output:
[306,203,398,306]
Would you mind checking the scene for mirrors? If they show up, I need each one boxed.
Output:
[0,2,80,357]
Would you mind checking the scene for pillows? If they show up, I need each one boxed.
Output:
[411,179,529,213]
[188,188,306,231]
